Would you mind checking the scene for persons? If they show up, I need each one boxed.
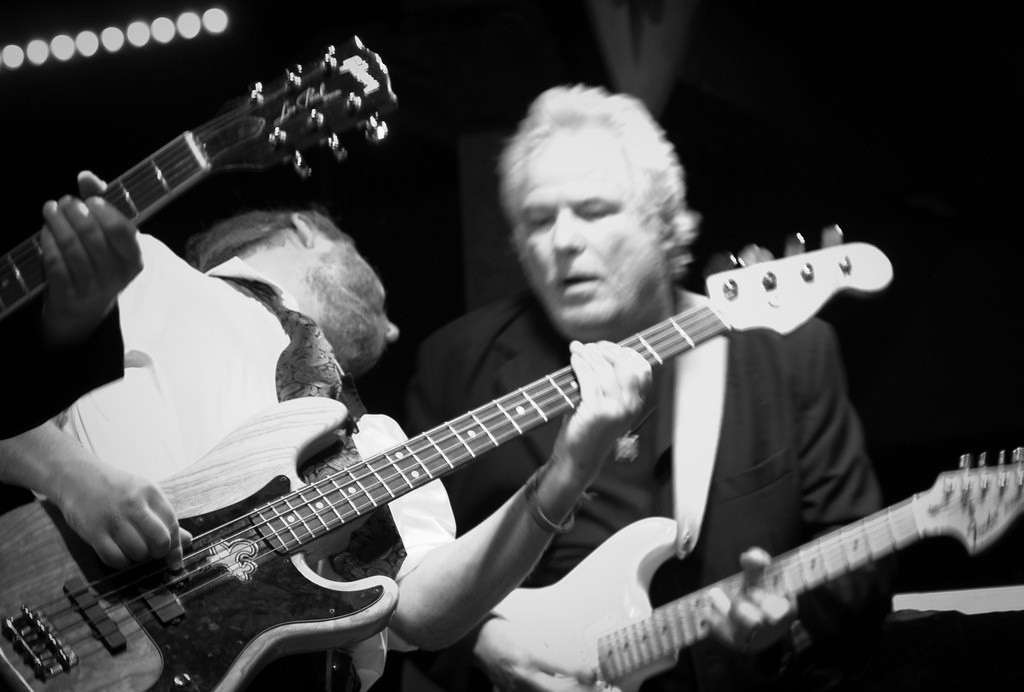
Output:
[0,83,894,692]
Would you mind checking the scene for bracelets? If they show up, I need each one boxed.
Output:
[526,470,598,535]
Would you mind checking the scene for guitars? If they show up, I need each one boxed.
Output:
[0,224,895,692]
[488,446,1024,692]
[0,34,399,322]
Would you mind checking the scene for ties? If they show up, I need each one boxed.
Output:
[218,276,407,581]
